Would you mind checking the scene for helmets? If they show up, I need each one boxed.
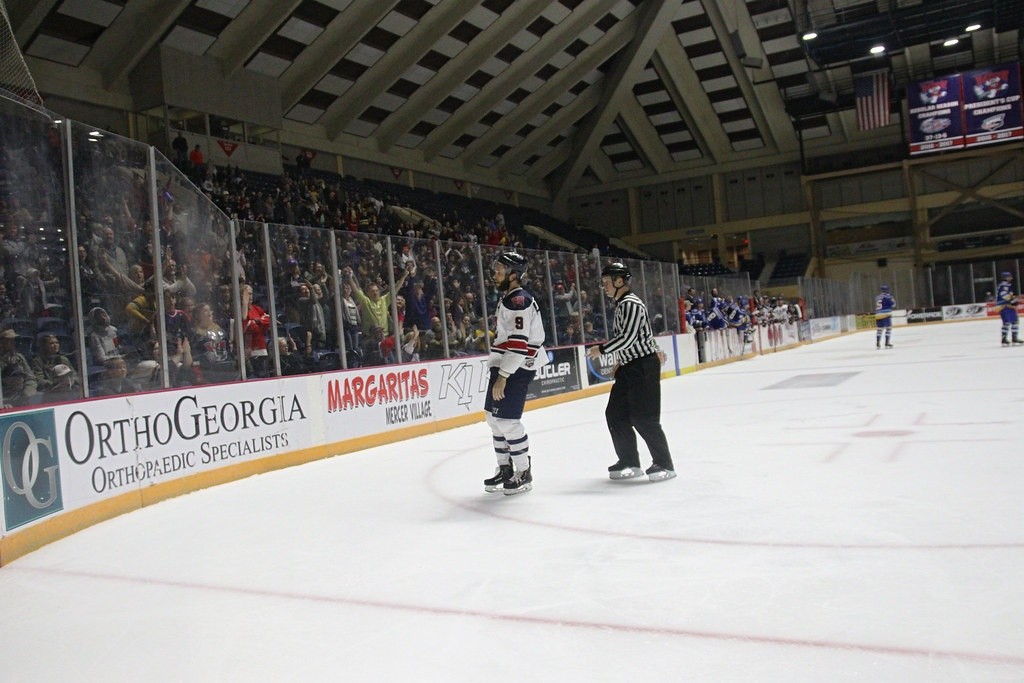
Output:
[498,251,527,280]
[601,262,632,286]
[880,284,890,292]
[1000,271,1012,282]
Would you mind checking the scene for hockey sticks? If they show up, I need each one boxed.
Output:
[772,324,777,353]
[757,313,764,355]
[743,328,756,356]
[724,329,732,353]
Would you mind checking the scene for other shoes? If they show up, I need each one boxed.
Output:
[749,326,757,334]
[743,335,753,344]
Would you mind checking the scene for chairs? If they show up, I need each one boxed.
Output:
[0,163,340,389]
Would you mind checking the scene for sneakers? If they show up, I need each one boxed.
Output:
[502,456,533,496]
[607,459,643,478]
[645,462,677,482]
[483,464,514,493]
[876,344,881,350]
[885,343,893,349]
[1001,339,1010,347]
[1011,337,1024,346]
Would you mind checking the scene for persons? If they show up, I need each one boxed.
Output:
[0,104,805,408]
[875,284,896,347]
[997,272,1024,345]
[586,262,675,475]
[483,251,551,490]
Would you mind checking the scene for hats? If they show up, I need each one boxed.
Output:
[0,329,21,340]
[1,363,33,378]
[767,297,777,303]
[739,299,748,306]
[777,296,785,300]
[737,296,744,300]
[683,299,694,306]
[369,324,384,336]
[288,257,298,266]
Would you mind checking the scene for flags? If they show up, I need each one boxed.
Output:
[851,55,892,131]
